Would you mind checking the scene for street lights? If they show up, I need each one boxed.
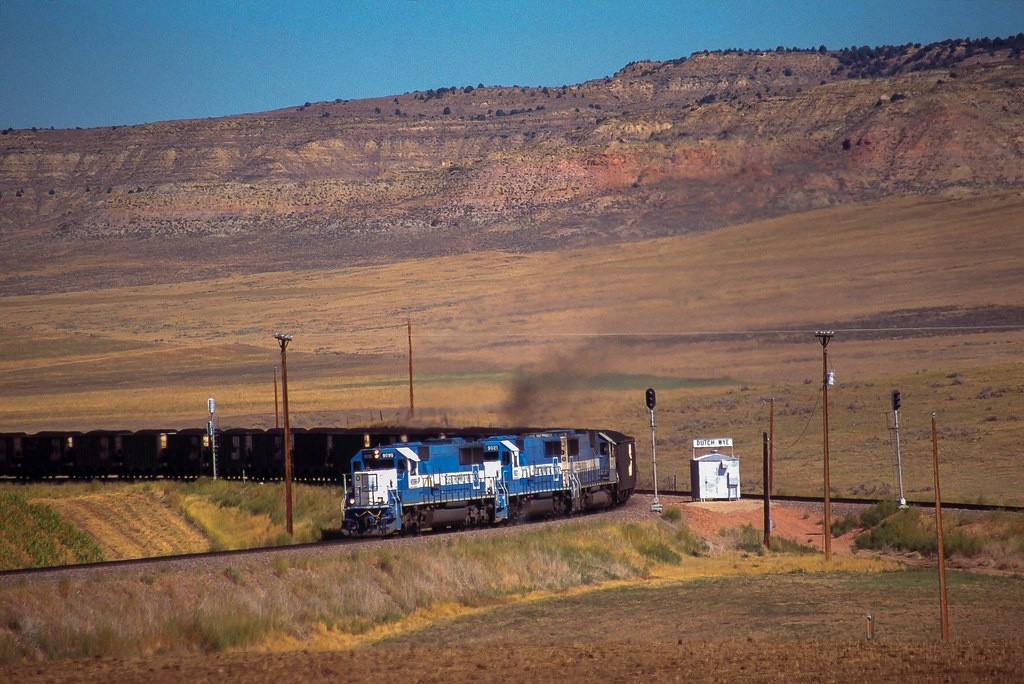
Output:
[275,333,296,537]
[814,330,835,559]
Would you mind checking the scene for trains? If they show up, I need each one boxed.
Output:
[0,428,639,538]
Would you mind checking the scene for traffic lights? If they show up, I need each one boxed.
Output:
[646,388,657,408]
[891,390,901,410]
